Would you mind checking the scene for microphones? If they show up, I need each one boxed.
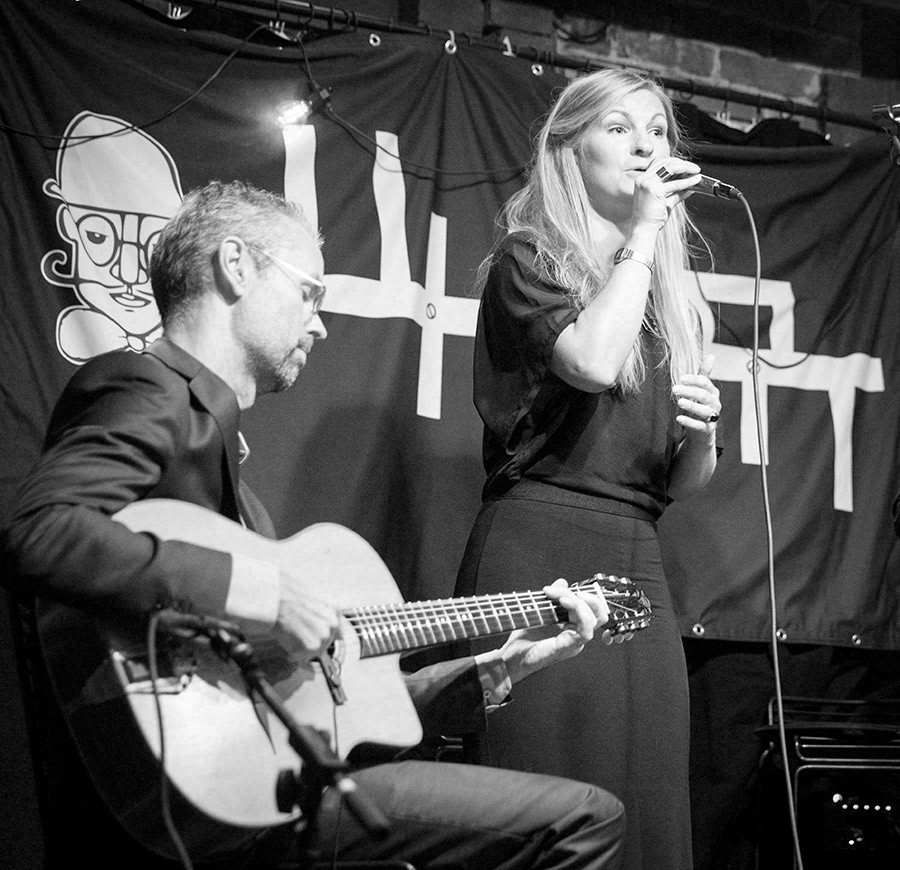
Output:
[667,172,743,200]
[155,612,246,647]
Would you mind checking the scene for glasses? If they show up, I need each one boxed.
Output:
[245,244,326,314]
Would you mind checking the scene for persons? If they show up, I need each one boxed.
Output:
[451,68,723,870]
[0,180,627,870]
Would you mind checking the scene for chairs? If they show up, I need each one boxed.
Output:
[0,529,418,870]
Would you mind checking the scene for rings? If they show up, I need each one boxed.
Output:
[705,412,719,423]
[656,167,672,183]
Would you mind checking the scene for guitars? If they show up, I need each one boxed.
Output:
[32,497,656,831]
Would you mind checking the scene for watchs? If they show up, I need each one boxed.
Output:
[612,248,655,274]
